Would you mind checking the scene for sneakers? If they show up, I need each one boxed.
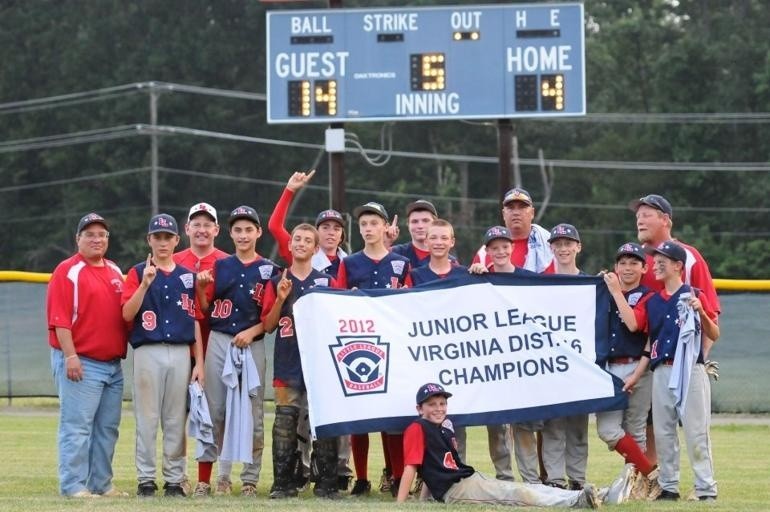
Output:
[102,487,131,496]
[605,463,636,506]
[136,481,157,497]
[70,488,105,498]
[197,482,212,497]
[345,479,371,498]
[214,479,232,496]
[579,482,601,508]
[647,464,664,501]
[162,483,184,497]
[240,484,259,499]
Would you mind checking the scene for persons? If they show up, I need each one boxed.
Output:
[173,204,232,497]
[396,384,635,508]
[384,200,460,270]
[119,214,206,498]
[623,241,717,502]
[470,189,556,276]
[260,224,342,495]
[627,194,722,360]
[197,205,283,498]
[46,212,129,500]
[596,243,662,503]
[403,221,489,466]
[268,170,355,491]
[483,225,544,485]
[337,201,413,498]
[540,223,593,490]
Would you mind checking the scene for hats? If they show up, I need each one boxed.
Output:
[404,199,437,217]
[415,383,453,404]
[78,213,110,236]
[644,241,686,263]
[484,225,512,246]
[546,224,579,244]
[503,189,531,207]
[315,210,345,230]
[229,206,261,229]
[627,194,672,218]
[148,213,178,237]
[353,203,388,223]
[186,202,218,224]
[614,243,645,264]
